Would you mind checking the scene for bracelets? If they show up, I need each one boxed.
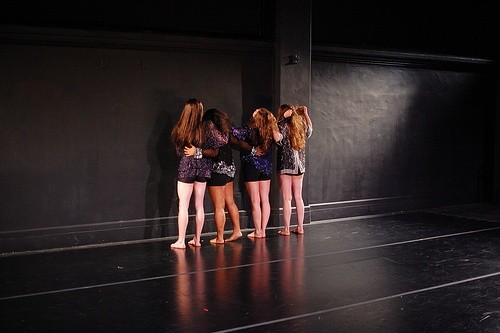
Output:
[250,145,258,157]
[194,148,202,158]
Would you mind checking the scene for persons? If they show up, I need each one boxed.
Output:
[267,104,313,235]
[184,108,264,244]
[168,97,230,249]
[229,107,285,237]
